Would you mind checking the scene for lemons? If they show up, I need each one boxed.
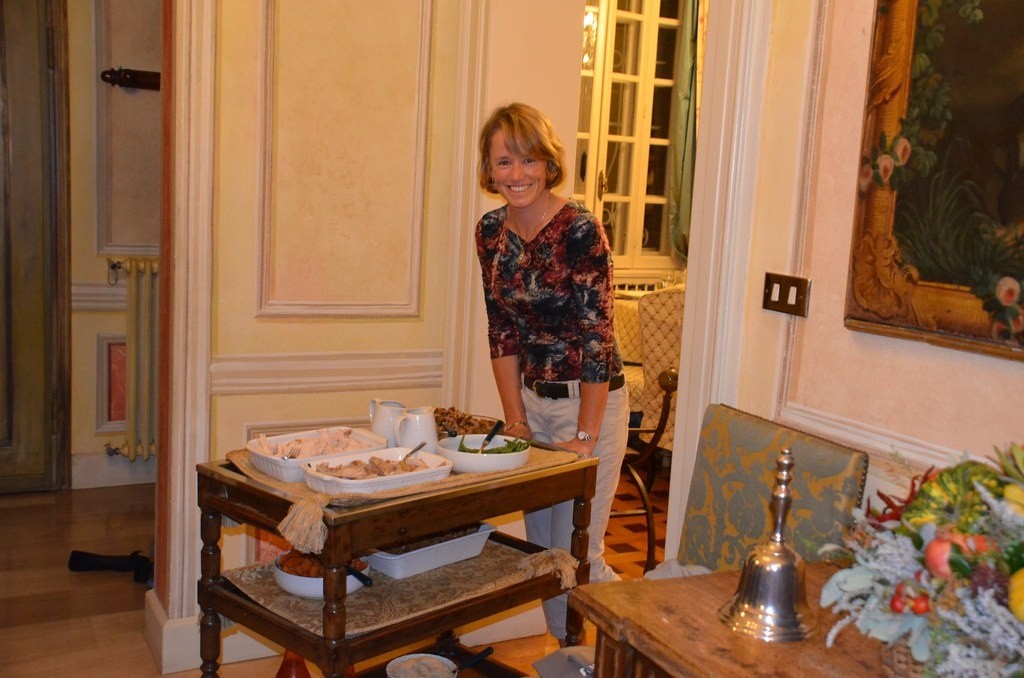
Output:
[1010,568,1024,622]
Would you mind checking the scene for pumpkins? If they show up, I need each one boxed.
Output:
[902,459,1024,546]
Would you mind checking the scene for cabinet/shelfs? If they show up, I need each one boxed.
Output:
[197,434,598,678]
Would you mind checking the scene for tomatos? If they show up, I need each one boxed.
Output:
[892,568,929,614]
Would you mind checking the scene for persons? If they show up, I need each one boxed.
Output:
[475,102,630,647]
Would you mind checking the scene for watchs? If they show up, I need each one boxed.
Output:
[575,430,599,442]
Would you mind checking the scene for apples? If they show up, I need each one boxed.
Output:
[927,534,1000,577]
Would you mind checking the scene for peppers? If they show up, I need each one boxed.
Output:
[865,466,935,532]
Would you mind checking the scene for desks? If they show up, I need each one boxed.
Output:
[563,562,899,678]
[614,296,645,410]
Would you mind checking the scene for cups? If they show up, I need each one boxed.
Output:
[386,654,459,678]
[369,399,408,448]
[662,272,676,288]
[393,406,438,453]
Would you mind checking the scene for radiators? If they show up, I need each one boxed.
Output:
[107,257,158,463]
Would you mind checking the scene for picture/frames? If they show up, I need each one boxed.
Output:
[844,1,1023,360]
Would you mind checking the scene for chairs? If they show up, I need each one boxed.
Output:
[533,400,869,678]
[639,284,686,493]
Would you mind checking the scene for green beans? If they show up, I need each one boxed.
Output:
[458,435,537,453]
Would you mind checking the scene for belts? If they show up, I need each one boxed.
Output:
[524,374,625,400]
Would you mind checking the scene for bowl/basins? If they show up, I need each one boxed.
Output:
[272,551,370,600]
[438,415,505,440]
[367,520,498,580]
[245,426,387,483]
[436,434,531,474]
[298,447,454,495]
